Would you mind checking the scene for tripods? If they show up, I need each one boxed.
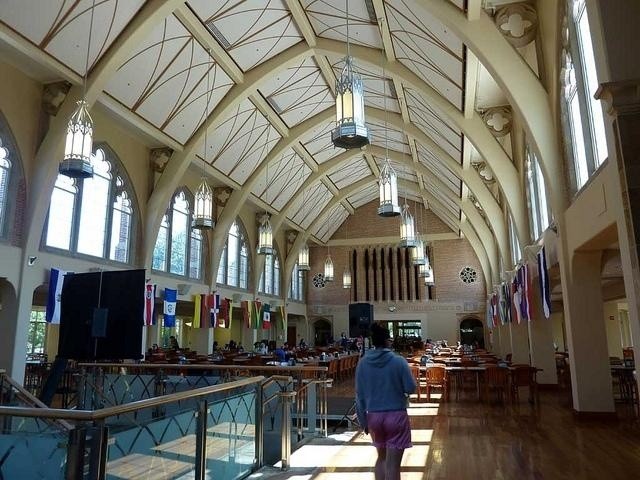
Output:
[333,338,365,431]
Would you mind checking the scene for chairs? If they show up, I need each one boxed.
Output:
[406,343,540,410]
[303,354,359,385]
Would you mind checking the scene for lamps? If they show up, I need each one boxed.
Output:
[191,45,215,231]
[323,185,335,282]
[58,1,94,180]
[331,0,370,151]
[256,114,275,256]
[377,14,436,287]
[297,155,312,271]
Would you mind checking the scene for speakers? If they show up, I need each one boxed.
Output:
[349,302,374,337]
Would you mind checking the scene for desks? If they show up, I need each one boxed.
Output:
[417,365,545,407]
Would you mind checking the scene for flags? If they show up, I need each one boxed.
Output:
[44,266,74,325]
[139,282,288,331]
[481,246,555,332]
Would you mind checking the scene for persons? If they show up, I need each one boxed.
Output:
[354,320,418,480]
[156,329,436,367]
